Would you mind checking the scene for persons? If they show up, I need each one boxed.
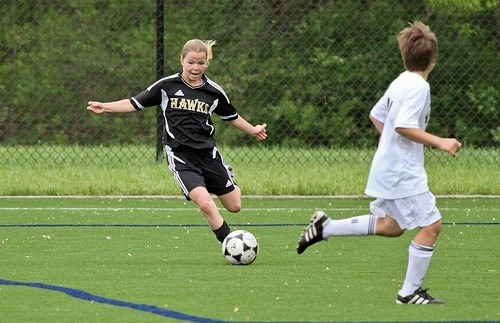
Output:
[87,38,266,244]
[296,19,462,304]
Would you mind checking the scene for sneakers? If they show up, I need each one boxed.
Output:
[296,210,331,254]
[396,286,445,304]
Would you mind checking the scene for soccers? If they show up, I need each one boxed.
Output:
[221,229,258,265]
